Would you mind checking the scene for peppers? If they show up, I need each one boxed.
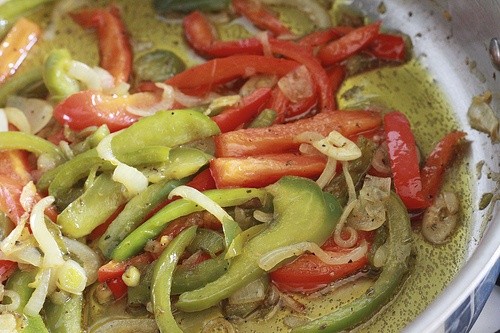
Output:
[0,0,467,333]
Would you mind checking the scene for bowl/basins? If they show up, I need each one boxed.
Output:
[339,0,500,333]
